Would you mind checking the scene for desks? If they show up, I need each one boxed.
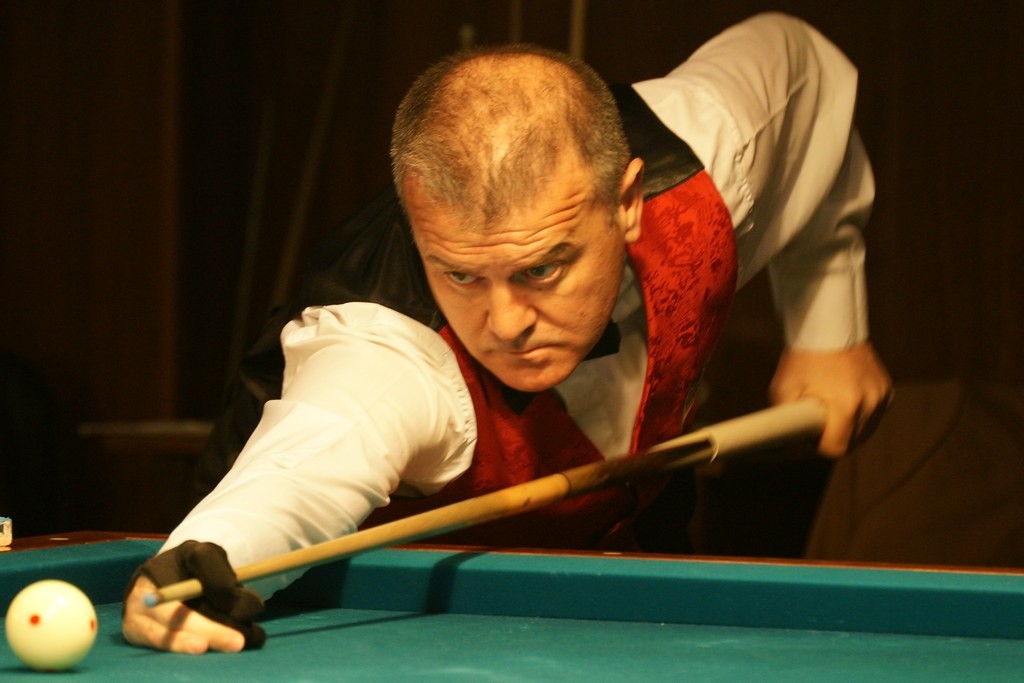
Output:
[78,415,217,531]
[0,530,1024,683]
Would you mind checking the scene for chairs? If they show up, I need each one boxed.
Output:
[0,357,86,539]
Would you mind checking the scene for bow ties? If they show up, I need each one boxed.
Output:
[501,316,621,418]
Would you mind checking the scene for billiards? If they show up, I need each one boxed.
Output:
[5,579,100,671]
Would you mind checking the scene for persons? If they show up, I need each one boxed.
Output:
[121,14,902,654]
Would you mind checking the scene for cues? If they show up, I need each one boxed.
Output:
[145,399,829,608]
